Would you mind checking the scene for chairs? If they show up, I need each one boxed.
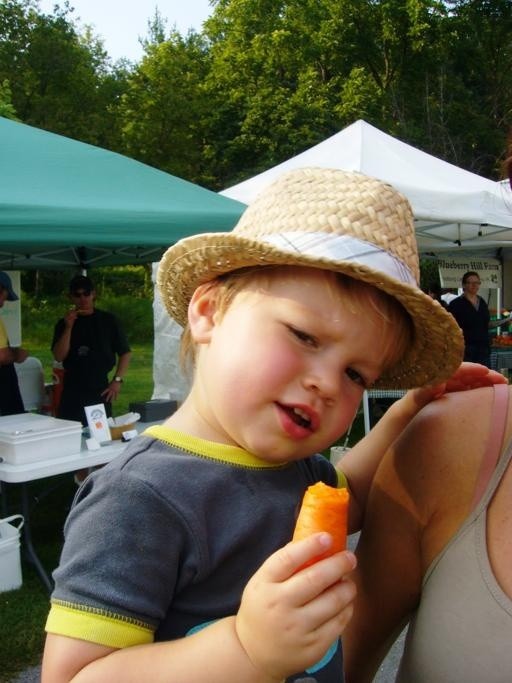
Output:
[13,356,52,416]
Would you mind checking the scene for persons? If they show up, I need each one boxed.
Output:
[49,274,134,429]
[343,354,512,682]
[35,164,510,683]
[446,272,493,375]
[1,270,31,416]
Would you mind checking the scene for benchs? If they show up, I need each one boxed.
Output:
[0,418,168,592]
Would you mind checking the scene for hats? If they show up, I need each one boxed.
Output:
[69,276,95,290]
[159,167,465,390]
[0,271,19,301]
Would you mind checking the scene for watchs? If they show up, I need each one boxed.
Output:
[113,376,123,384]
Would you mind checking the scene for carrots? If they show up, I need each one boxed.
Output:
[291,479,350,582]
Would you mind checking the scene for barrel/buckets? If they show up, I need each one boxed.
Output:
[0,515,25,594]
[51,361,66,405]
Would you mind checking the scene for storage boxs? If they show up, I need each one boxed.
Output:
[0,411,84,465]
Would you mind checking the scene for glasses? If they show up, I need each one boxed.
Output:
[71,290,93,297]
